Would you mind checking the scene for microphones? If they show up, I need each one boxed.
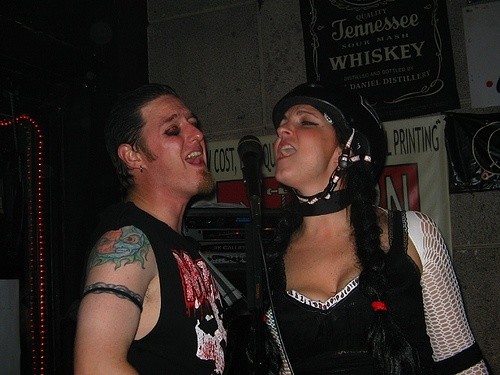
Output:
[238,133,263,231]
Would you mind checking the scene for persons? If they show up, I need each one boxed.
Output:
[71,82,235,375]
[230,81,490,374]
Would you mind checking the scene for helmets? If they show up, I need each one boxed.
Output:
[273,82,388,184]
